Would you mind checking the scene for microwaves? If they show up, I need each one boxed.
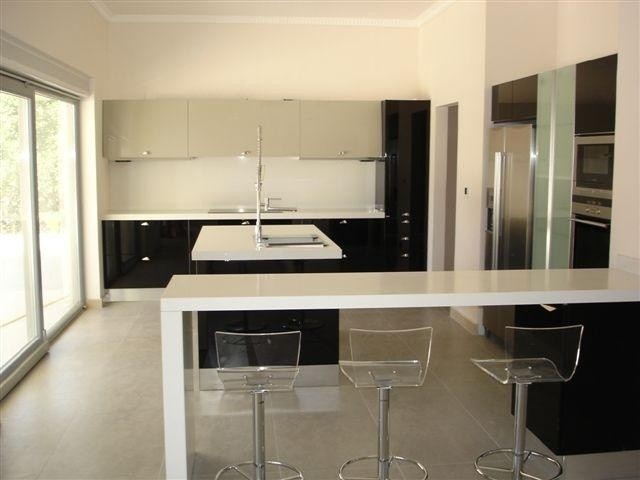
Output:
[573,142,612,201]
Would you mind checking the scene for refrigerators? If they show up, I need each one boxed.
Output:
[483,121,536,344]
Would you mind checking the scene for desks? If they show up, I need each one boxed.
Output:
[157,263,640,480]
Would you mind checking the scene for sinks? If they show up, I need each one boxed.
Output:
[208,207,298,213]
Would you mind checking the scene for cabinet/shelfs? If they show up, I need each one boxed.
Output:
[491,73,537,124]
[189,97,301,158]
[101,219,189,302]
[375,100,430,272]
[102,98,188,161]
[301,99,383,161]
[302,218,388,272]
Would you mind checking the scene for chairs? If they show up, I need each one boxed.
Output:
[467,324,587,480]
[338,326,434,479]
[214,329,304,479]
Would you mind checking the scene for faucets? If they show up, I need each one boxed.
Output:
[260,197,282,211]
